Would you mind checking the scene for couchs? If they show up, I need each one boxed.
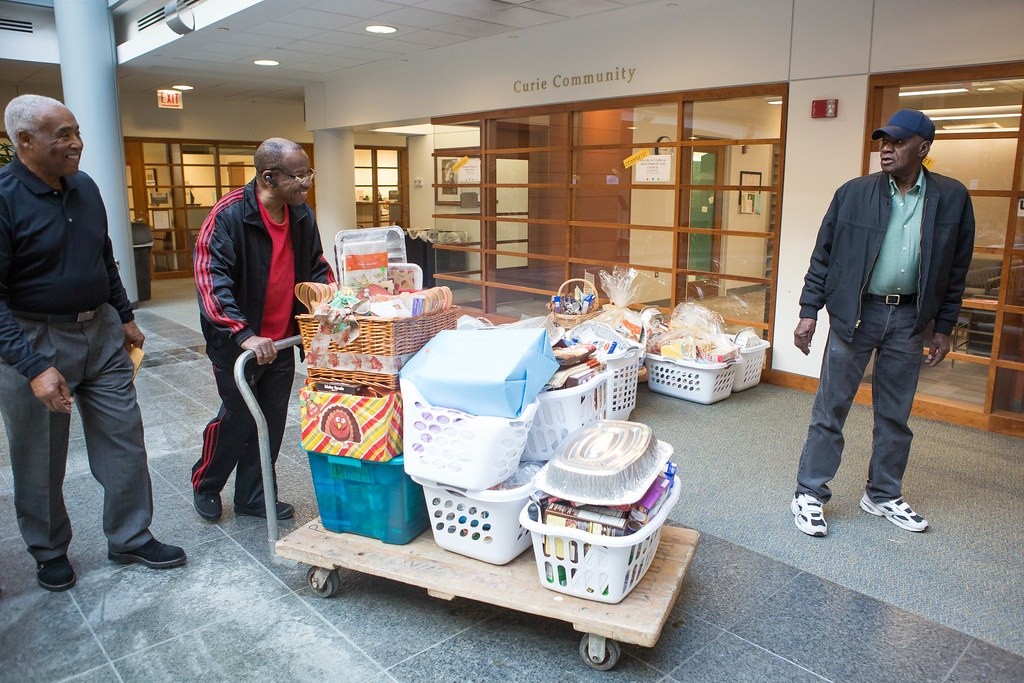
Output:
[963,257,1024,353]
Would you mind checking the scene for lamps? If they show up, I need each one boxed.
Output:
[899,83,972,97]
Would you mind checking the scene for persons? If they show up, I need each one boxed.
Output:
[0,94,189,590]
[790,107,976,537]
[189,138,335,525]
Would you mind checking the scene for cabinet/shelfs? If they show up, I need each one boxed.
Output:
[763,142,779,341]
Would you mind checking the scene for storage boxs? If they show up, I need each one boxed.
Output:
[298,224,772,600]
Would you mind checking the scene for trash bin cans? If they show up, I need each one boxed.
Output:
[436,230,466,287]
[405,228,435,287]
[131,222,154,302]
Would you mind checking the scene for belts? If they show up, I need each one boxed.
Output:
[11,302,102,323]
[862,292,917,306]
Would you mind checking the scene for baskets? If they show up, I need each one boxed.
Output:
[521,374,612,460]
[399,377,540,490]
[410,475,548,565]
[637,366,646,383]
[734,328,761,348]
[295,306,460,391]
[645,352,743,404]
[519,475,682,603]
[597,355,638,421]
[551,278,608,328]
[726,335,771,392]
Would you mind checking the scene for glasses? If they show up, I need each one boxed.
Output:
[271,168,316,190]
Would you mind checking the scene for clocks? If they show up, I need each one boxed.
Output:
[655,136,674,158]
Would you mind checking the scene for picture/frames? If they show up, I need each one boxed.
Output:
[738,171,763,206]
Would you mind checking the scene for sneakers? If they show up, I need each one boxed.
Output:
[860,490,928,531]
[38,554,77,591]
[107,537,186,568]
[193,488,224,521]
[234,501,294,519]
[791,492,827,536]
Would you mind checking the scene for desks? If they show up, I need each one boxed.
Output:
[950,295,999,367]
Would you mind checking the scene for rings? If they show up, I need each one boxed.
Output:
[263,352,269,355]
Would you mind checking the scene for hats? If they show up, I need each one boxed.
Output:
[872,108,936,145]
